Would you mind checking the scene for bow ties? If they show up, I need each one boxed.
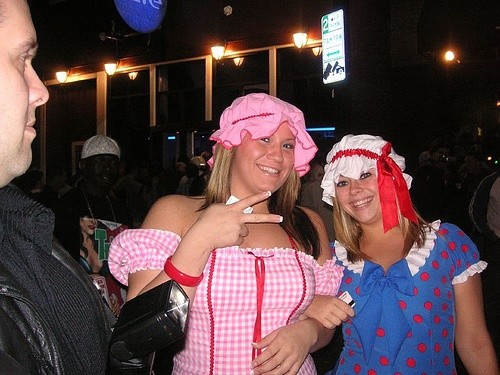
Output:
[352,257,416,369]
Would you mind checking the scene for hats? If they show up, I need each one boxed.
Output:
[206,92,318,177]
[81,134,120,160]
[320,133,420,233]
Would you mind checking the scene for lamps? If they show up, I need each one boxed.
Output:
[128,46,323,80]
[55,31,307,86]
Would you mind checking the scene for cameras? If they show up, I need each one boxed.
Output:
[108,279,190,375]
